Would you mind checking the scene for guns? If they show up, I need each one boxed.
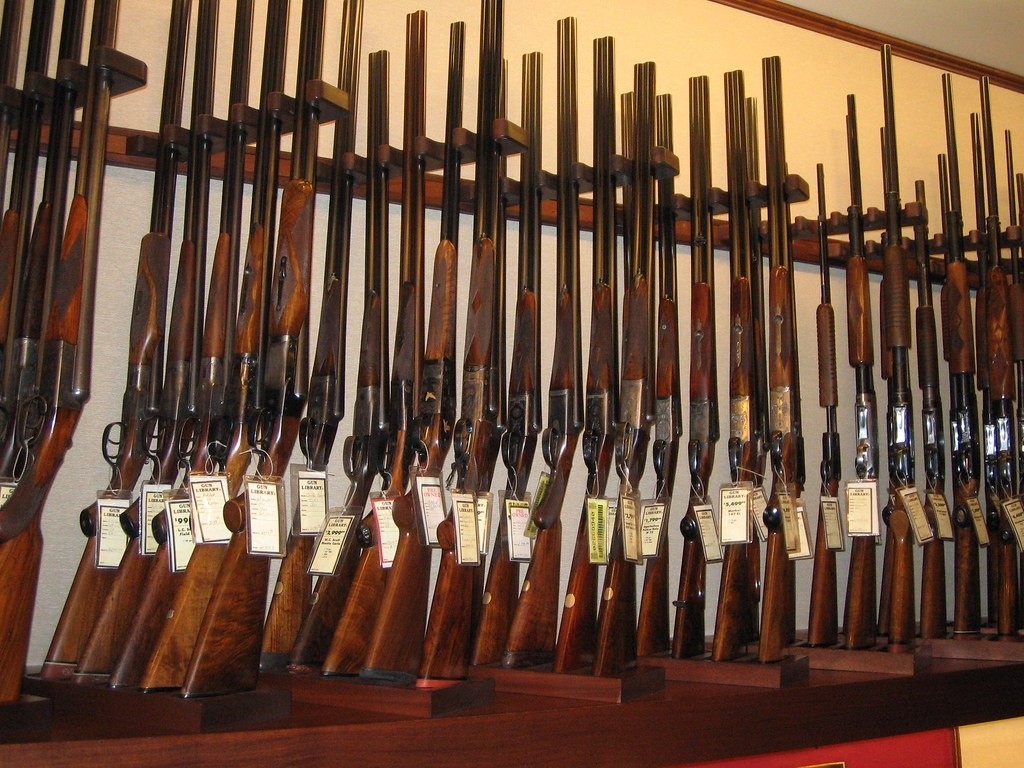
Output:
[0,1,1023,710]
